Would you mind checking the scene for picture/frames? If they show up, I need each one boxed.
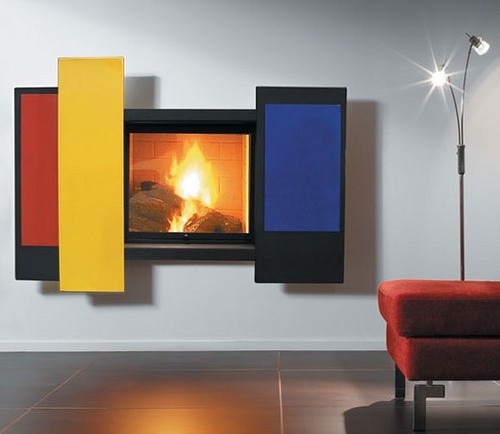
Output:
[123,107,257,261]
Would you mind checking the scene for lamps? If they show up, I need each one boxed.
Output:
[425,33,490,282]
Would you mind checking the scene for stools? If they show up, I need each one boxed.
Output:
[377,280,500,434]
